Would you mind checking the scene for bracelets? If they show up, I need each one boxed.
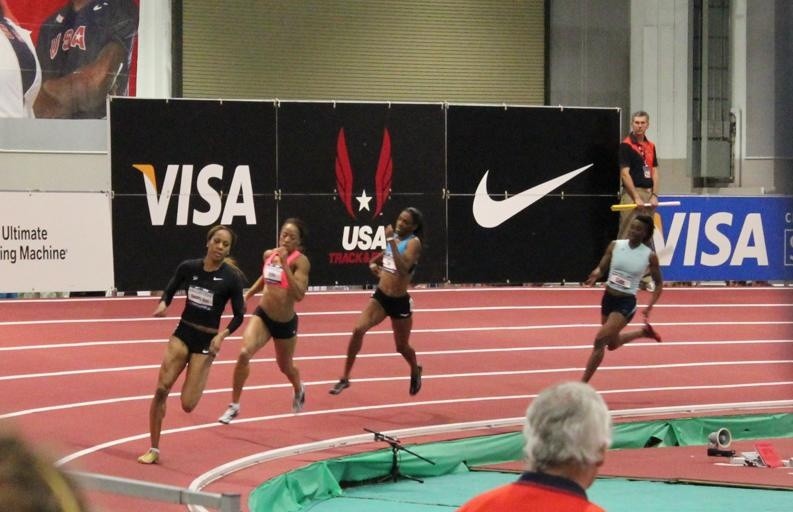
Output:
[385,237,394,242]
[652,193,658,198]
[368,263,379,271]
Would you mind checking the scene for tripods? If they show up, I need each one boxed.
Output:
[378,447,426,485]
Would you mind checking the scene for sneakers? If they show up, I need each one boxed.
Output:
[329,379,350,394]
[642,323,662,342]
[218,404,241,424]
[138,450,160,464]
[292,384,306,412]
[409,363,423,395]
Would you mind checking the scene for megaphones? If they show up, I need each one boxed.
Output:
[708,428,732,448]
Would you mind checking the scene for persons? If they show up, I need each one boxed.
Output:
[330,206,424,396]
[617,111,660,290]
[138,224,244,465]
[454,382,610,511]
[581,215,663,382]
[218,219,311,424]
[0,0,139,119]
